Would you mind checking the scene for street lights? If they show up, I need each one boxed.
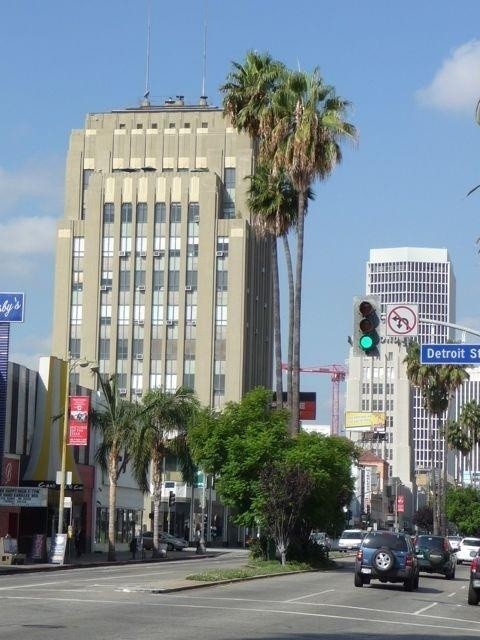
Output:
[57,355,100,532]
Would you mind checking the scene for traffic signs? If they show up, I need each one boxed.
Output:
[420,343,480,365]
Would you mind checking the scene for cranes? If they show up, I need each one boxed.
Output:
[281,362,345,436]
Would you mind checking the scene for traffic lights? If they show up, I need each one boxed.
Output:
[169,491,176,507]
[353,294,381,358]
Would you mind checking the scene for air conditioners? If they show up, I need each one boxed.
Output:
[119,388,127,394]
[100,251,222,361]
[135,389,142,394]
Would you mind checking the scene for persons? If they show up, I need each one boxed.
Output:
[72,524,86,558]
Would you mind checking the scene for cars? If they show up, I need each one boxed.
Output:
[143,531,189,551]
[308,532,331,552]
[338,529,479,605]
